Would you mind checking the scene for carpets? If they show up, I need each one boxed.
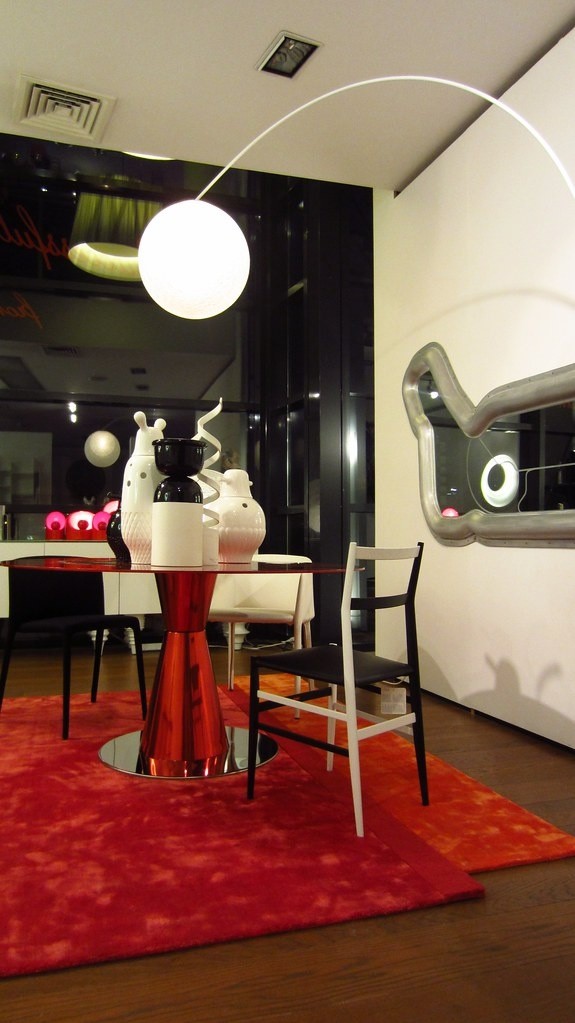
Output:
[0,674,575,979]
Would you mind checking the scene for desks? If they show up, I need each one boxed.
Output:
[0,557,365,779]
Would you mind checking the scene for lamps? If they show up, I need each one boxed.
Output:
[138,76,575,321]
[83,415,120,466]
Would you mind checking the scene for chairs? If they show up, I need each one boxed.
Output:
[247,542,429,837]
[0,555,146,740]
[207,554,315,719]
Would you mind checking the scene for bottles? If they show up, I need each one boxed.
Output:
[203,469,266,563]
[121,411,167,564]
